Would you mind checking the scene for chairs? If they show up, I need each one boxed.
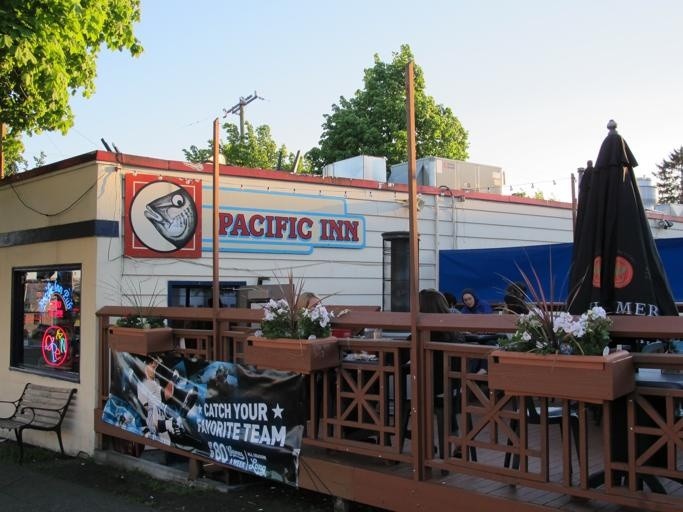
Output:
[392,334,580,488]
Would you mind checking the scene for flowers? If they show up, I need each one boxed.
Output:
[489,243,615,357]
[252,266,339,342]
[98,272,165,329]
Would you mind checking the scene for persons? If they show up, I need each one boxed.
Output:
[147,365,232,442]
[443,279,529,385]
[137,353,181,447]
[297,289,351,362]
[375,287,462,460]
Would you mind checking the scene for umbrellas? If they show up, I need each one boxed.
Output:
[566,158,598,295]
[566,116,680,352]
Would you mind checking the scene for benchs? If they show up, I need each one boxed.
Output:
[0,383,76,465]
